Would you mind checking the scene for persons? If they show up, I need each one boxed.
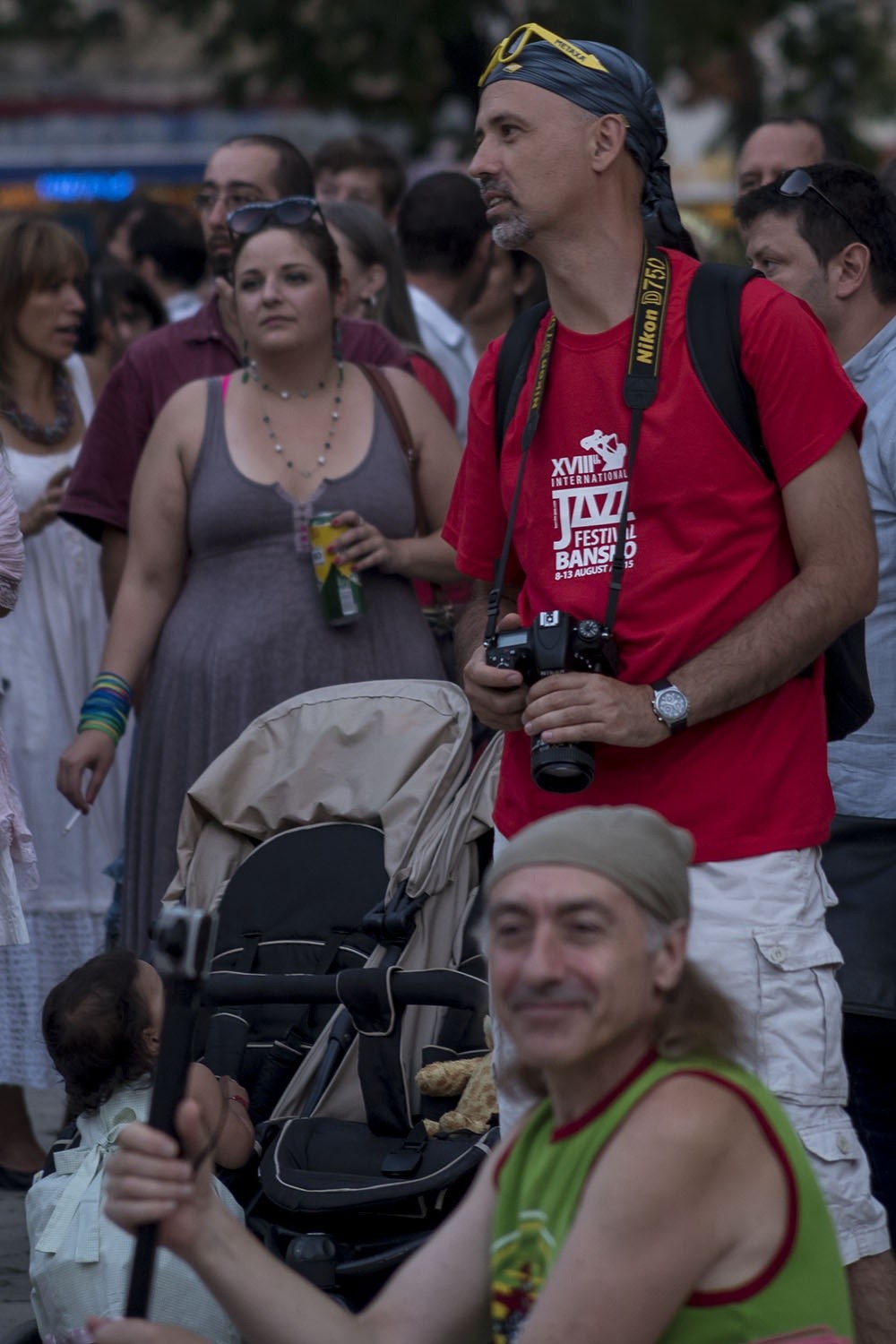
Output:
[733,111,875,199]
[0,437,51,948]
[732,162,896,1254]
[440,22,896,1344]
[54,134,418,679]
[24,947,256,1344]
[55,195,464,953]
[0,218,136,1190]
[82,804,853,1344]
[22,134,542,437]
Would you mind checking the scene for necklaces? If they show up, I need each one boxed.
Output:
[0,363,77,444]
[251,360,344,479]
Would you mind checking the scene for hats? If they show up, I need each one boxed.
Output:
[483,802,696,934]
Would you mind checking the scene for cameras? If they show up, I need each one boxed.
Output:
[485,609,615,794]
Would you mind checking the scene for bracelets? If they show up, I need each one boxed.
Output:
[76,671,133,747]
[226,1095,248,1110]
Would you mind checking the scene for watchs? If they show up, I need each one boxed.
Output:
[651,678,691,734]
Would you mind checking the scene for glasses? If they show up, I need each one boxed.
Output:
[226,198,333,240]
[779,169,864,239]
[196,191,253,210]
[473,20,606,85]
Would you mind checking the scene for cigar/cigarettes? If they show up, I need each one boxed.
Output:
[62,809,82,837]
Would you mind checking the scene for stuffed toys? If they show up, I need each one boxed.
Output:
[414,1015,499,1139]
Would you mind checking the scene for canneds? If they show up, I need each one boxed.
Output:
[308,511,368,627]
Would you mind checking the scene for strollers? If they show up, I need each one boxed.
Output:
[146,674,507,1310]
